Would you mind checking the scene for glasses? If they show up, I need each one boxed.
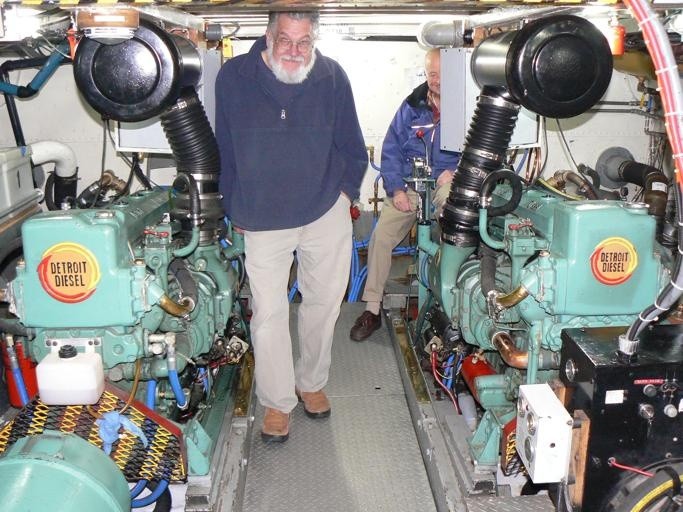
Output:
[276,38,312,51]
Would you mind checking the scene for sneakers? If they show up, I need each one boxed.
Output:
[261,407,290,442]
[350,309,381,341]
[295,385,331,419]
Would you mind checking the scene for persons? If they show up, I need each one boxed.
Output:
[211,8,371,446]
[345,46,463,343]
